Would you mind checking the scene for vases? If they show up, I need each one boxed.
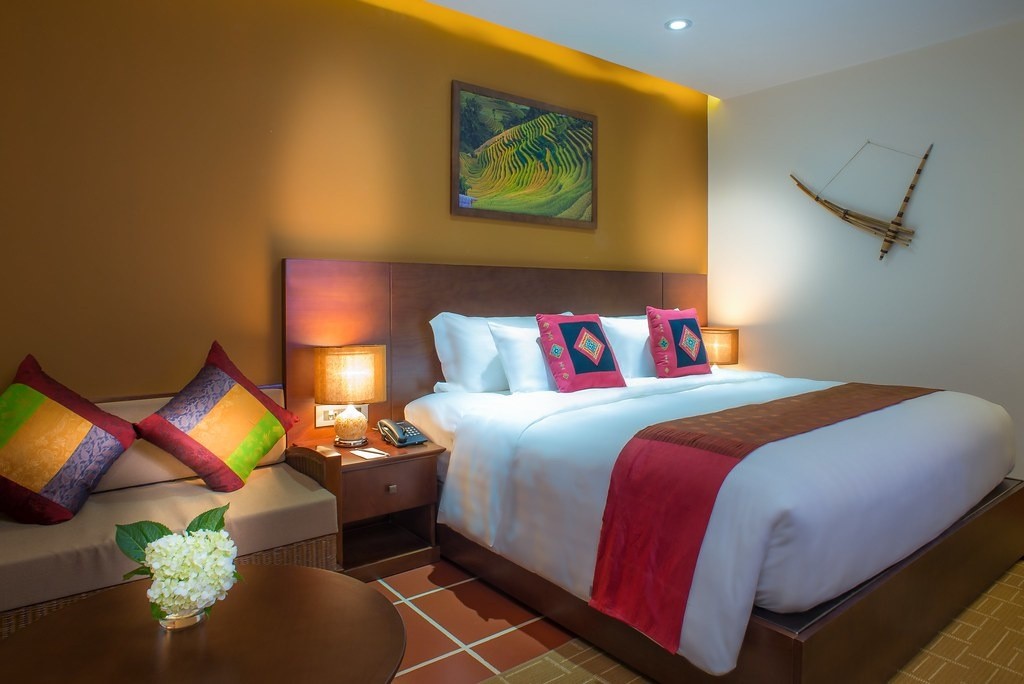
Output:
[159,608,208,631]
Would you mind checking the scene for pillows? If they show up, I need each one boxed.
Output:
[135,340,300,491]
[428,311,575,394]
[599,308,681,380]
[536,313,628,393]
[486,320,560,394]
[0,354,139,525]
[646,306,712,378]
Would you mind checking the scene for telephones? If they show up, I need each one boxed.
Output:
[376,418,428,448]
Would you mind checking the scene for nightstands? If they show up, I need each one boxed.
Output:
[317,426,446,584]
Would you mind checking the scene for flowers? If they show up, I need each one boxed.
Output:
[108,503,244,622]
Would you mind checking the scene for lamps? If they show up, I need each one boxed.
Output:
[699,327,739,369]
[313,344,388,448]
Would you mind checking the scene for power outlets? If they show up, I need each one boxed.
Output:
[315,404,369,428]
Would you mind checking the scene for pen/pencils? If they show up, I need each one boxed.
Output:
[354,448,386,455]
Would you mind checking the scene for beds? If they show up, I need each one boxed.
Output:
[279,254,1024,684]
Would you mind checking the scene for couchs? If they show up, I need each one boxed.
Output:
[0,382,342,639]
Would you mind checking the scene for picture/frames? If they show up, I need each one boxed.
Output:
[451,79,599,231]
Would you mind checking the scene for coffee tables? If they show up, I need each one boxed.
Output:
[0,563,408,684]
